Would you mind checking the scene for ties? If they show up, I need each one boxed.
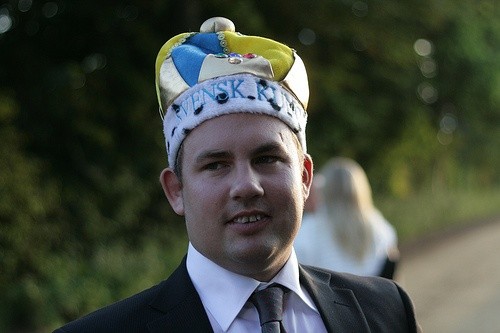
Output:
[248,285,291,333]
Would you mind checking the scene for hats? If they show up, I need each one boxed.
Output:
[153,17,310,169]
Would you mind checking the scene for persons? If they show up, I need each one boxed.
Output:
[52,17,424,333]
[292,156,398,276]
